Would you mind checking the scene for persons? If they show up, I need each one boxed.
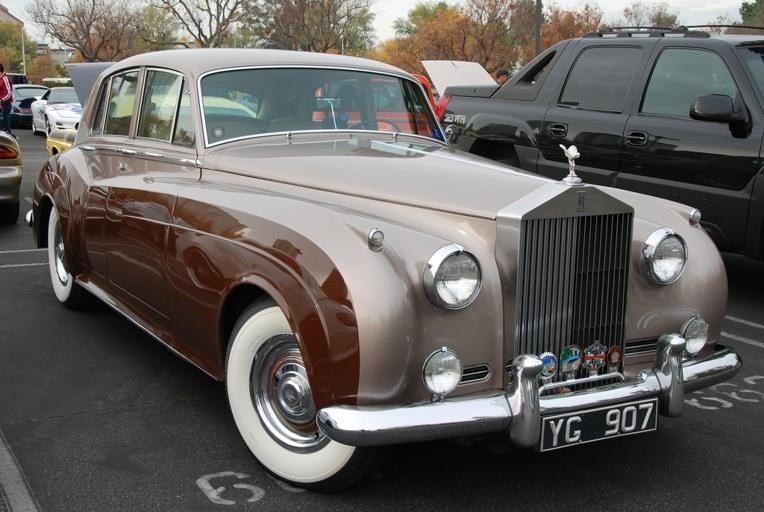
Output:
[0,65,20,141]
[493,69,514,86]
